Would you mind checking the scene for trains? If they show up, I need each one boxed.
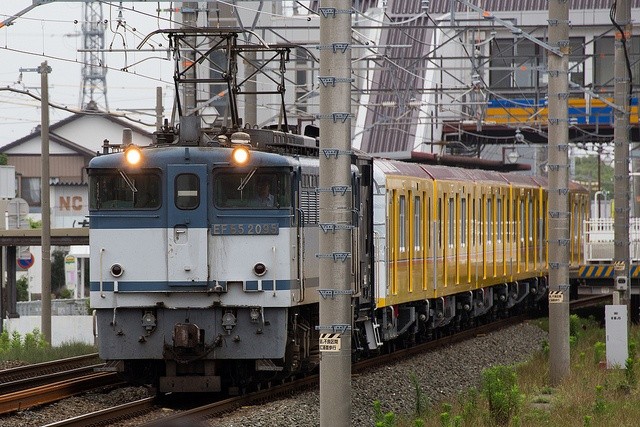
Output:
[86,146,592,409]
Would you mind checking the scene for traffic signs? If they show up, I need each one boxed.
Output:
[17,252,34,268]
